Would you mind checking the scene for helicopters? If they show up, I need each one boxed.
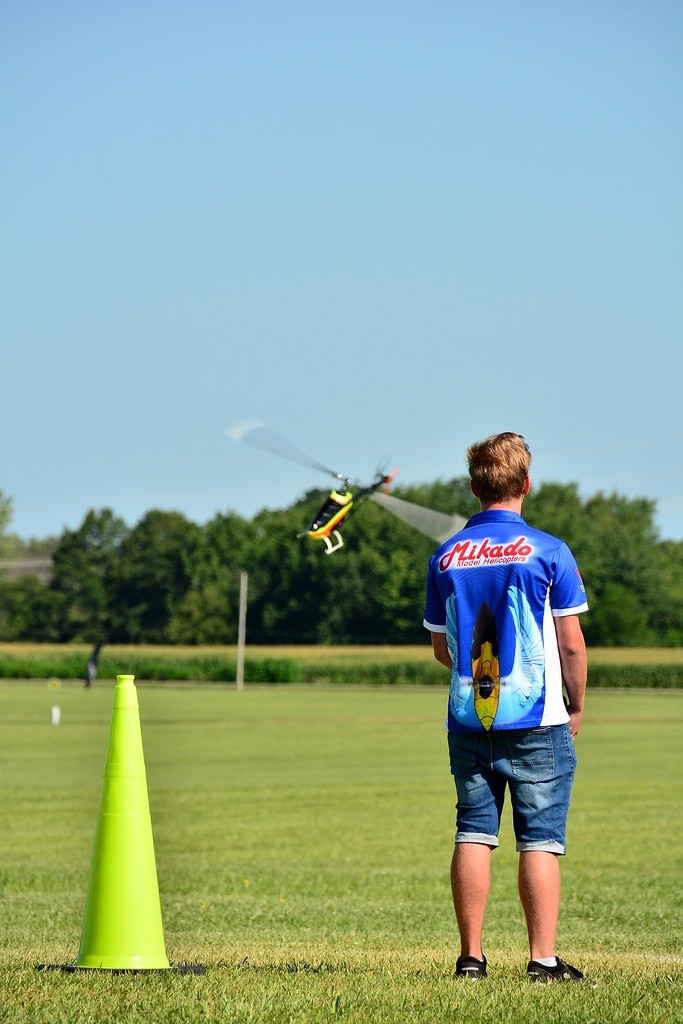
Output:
[257,439,471,556]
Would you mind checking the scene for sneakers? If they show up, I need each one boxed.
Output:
[527,955,597,988]
[454,954,488,978]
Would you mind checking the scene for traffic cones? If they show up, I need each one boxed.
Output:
[54,674,173,972]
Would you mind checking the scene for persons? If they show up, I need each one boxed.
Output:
[423,432,589,984]
[85,640,104,687]
